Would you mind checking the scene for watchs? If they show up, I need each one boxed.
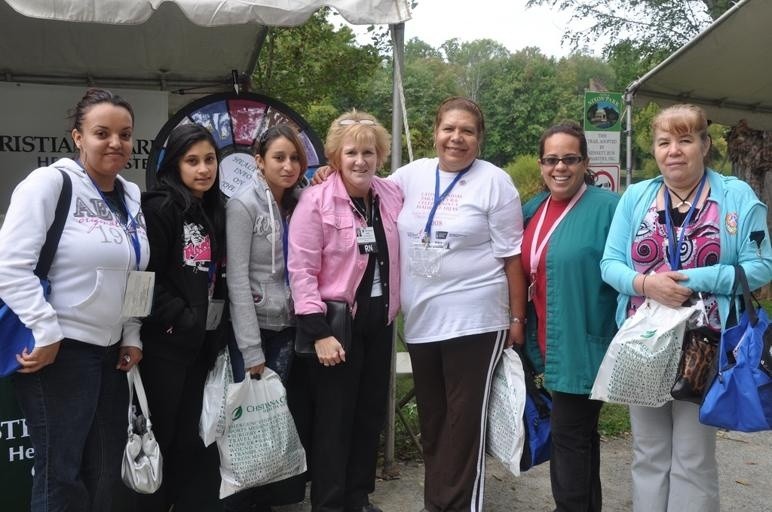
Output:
[509,317,529,325]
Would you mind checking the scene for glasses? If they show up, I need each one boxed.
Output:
[539,155,584,167]
[338,117,376,128]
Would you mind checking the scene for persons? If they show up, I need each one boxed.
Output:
[522,121,623,512]
[287,112,403,512]
[135,123,227,512]
[600,104,772,512]
[0,88,152,512]
[225,126,310,512]
[310,97,528,512]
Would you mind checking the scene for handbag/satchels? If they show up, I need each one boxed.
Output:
[696,261,771,435]
[212,362,311,501]
[670,323,726,408]
[196,338,236,450]
[585,296,699,410]
[291,295,355,362]
[482,342,527,479]
[0,161,76,382]
[115,351,168,498]
[510,341,558,472]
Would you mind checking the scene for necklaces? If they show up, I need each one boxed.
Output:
[347,186,370,223]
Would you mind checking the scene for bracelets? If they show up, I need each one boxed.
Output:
[642,273,649,299]
